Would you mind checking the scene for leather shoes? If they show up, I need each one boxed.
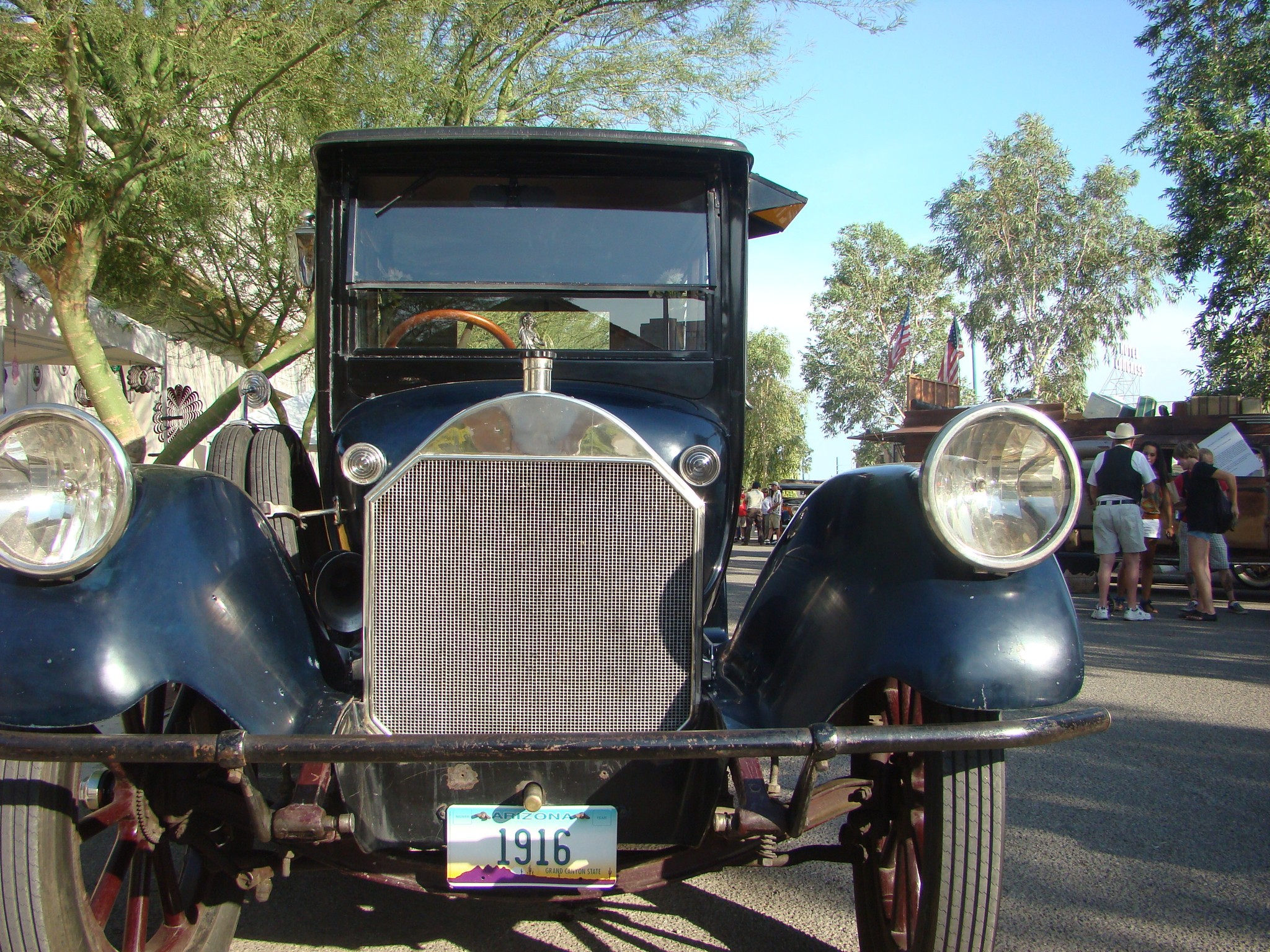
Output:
[1140,599,1158,613]
[1115,599,1124,610]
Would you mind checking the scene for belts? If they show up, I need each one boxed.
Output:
[1096,500,1137,506]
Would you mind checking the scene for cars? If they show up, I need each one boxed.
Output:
[0,122,1105,950]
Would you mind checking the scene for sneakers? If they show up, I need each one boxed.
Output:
[1228,602,1247,613]
[1181,601,1198,611]
[1124,605,1151,620]
[1091,604,1109,619]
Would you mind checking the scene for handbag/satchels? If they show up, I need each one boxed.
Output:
[1218,486,1233,534]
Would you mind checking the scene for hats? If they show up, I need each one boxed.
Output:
[769,481,778,486]
[1106,423,1144,439]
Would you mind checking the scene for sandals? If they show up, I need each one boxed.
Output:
[1178,609,1217,620]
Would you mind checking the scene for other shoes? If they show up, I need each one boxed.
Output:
[733,538,737,543]
[771,540,778,545]
[741,542,748,546]
[760,542,766,545]
[764,539,770,544]
[741,538,745,542]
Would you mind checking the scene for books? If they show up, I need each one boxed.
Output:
[1165,480,1181,505]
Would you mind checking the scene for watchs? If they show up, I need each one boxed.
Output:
[1168,524,1174,528]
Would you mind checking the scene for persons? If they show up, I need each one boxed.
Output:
[1086,423,1156,621]
[732,480,785,545]
[1175,440,1249,622]
[1113,440,1175,614]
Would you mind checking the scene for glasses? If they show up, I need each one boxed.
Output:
[741,489,744,491]
[763,491,768,493]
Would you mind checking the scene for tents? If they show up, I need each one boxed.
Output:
[0,273,168,450]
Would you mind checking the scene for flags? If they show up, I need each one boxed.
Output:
[938,309,965,386]
[881,297,911,383]
